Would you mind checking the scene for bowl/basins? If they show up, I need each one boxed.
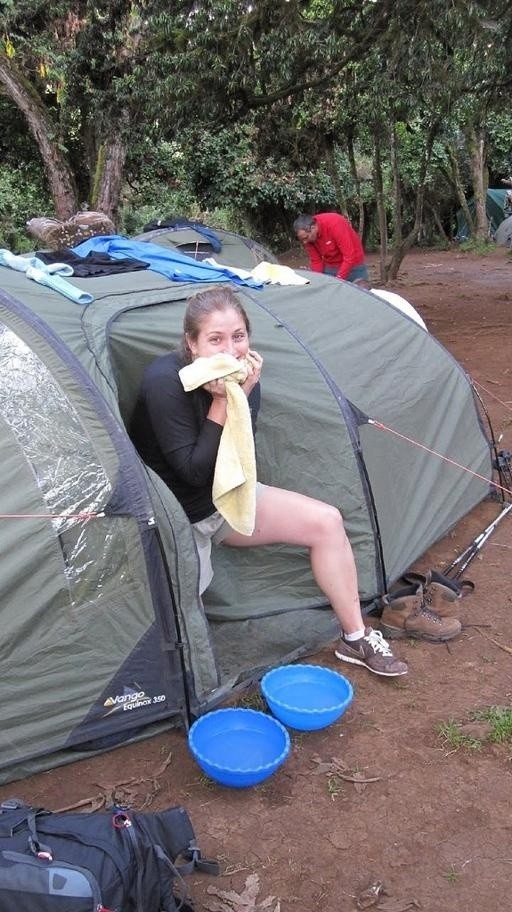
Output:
[188,665,353,787]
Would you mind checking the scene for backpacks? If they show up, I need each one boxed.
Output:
[0,800,197,911]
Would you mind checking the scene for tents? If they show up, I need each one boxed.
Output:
[127,223,283,272]
[492,216,511,244]
[0,217,503,787]
[446,189,512,244]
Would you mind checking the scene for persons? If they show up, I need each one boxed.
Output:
[126,286,410,679]
[292,212,370,290]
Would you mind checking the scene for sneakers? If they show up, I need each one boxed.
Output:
[333,625,409,678]
[423,565,460,619]
[378,581,462,642]
